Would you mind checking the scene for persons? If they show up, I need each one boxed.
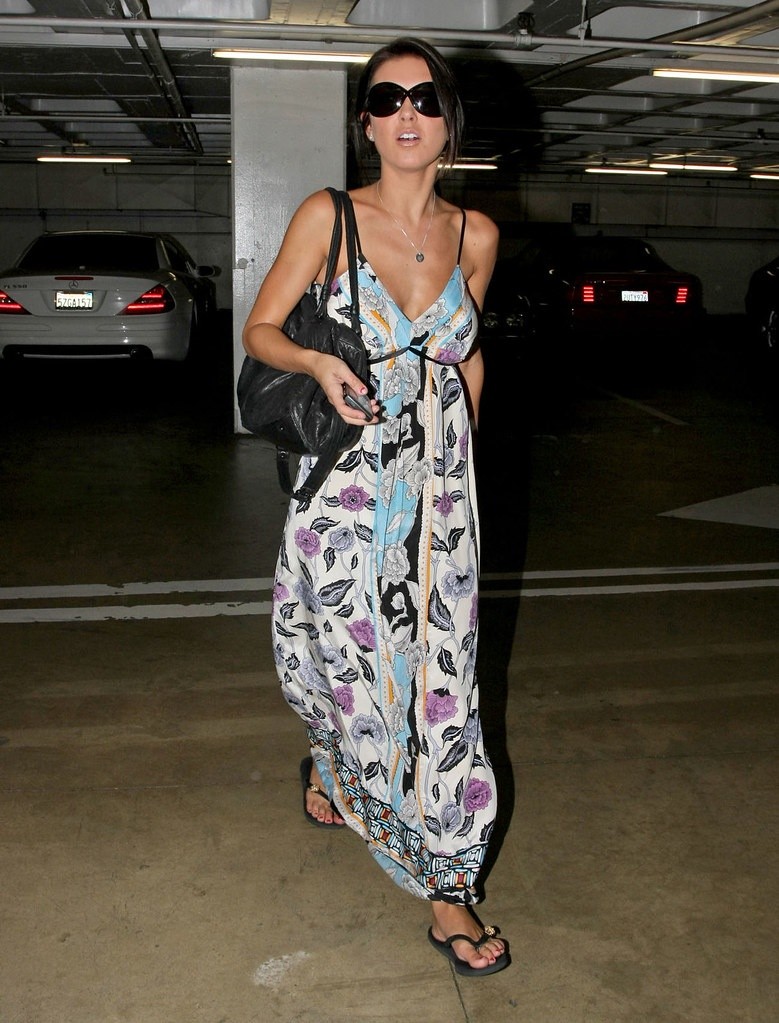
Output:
[243,35,507,969]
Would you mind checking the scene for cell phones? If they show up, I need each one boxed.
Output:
[343,382,373,423]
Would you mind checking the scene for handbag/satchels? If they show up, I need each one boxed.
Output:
[236,187,368,502]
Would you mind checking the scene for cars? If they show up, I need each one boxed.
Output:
[0,230,219,376]
[744,251,779,370]
[485,279,546,366]
[525,231,708,358]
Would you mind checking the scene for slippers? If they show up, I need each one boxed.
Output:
[428,925,508,977]
[300,757,346,829]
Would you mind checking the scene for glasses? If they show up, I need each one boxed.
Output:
[365,82,444,118]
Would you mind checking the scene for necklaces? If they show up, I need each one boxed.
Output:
[376,179,436,262]
